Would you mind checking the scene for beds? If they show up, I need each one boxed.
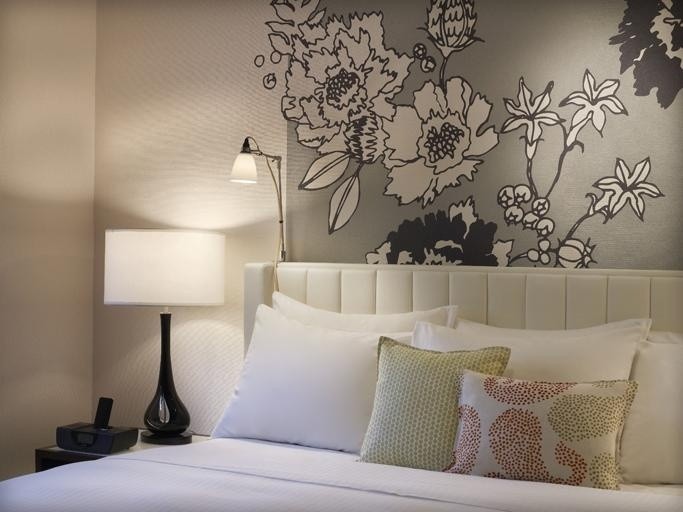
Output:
[0,263,680,512]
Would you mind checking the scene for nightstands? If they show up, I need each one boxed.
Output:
[32,440,135,473]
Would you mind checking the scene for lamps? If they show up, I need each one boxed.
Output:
[227,135,289,263]
[100,224,229,440]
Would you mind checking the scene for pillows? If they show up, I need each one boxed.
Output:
[454,318,653,340]
[354,336,511,472]
[272,290,459,333]
[621,340,682,488]
[410,320,645,382]
[210,303,414,455]
[441,368,639,491]
[646,329,682,345]
